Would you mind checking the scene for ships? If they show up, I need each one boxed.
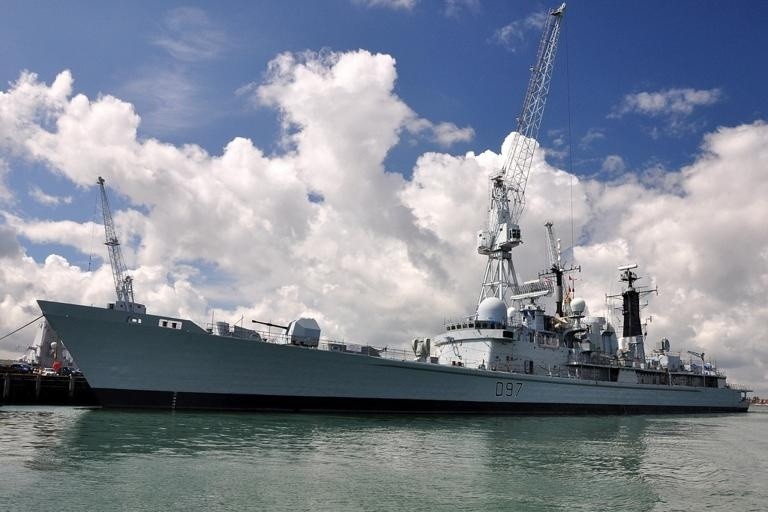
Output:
[36,221,754,418]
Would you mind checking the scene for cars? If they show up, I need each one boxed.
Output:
[12,363,84,377]
